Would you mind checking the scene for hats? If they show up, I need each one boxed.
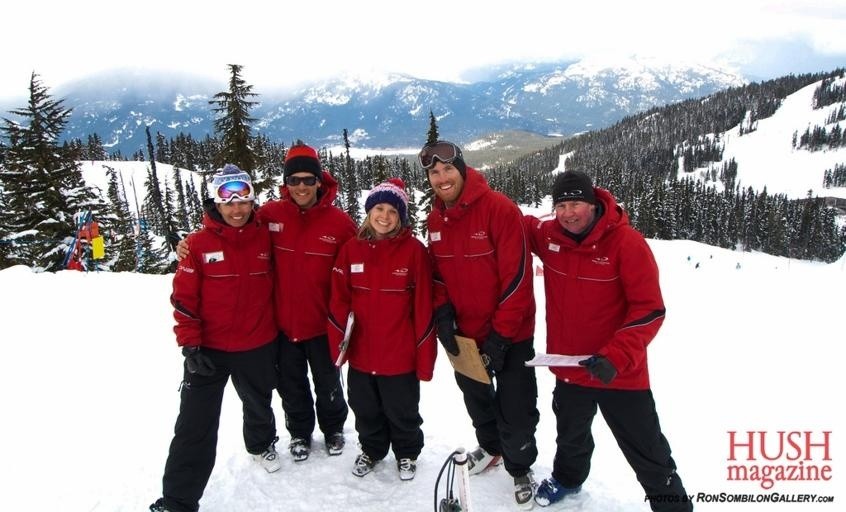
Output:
[282,143,324,184]
[213,162,255,205]
[364,176,410,226]
[551,169,597,208]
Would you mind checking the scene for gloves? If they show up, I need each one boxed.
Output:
[433,301,461,357]
[478,326,513,380]
[578,353,619,386]
[180,344,218,377]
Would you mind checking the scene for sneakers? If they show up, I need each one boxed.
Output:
[466,444,505,478]
[397,456,417,483]
[513,468,539,510]
[324,430,346,457]
[533,475,583,508]
[248,436,282,474]
[351,452,382,478]
[287,436,312,462]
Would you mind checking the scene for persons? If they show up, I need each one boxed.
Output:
[148,164,280,512]
[327,178,438,480]
[419,141,540,511]
[176,145,359,462]
[523,170,693,512]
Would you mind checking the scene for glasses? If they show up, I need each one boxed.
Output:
[215,179,255,205]
[284,175,319,186]
[417,141,464,170]
